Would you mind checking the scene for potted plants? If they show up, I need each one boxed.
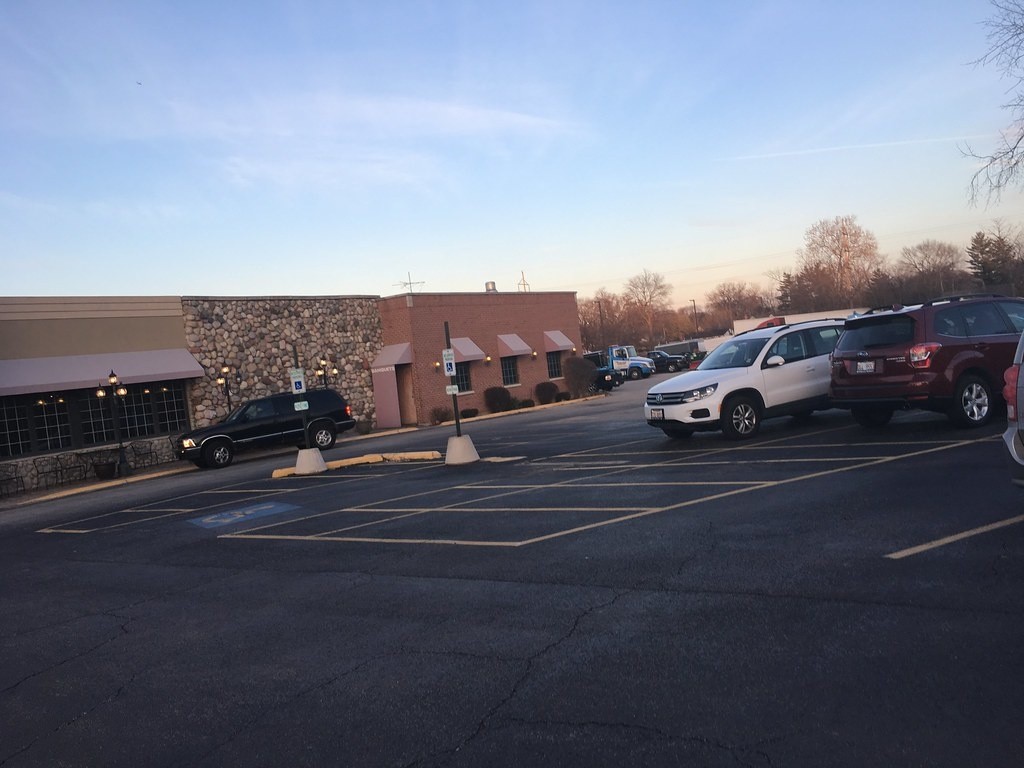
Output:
[354,400,377,435]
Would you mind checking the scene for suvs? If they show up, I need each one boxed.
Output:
[175,384,357,470]
[643,317,849,441]
[828,293,1024,427]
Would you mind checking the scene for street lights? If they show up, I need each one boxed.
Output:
[93,369,133,477]
[314,355,340,392]
[214,360,242,418]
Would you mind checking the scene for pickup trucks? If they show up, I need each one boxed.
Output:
[644,350,687,372]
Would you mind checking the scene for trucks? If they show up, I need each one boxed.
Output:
[635,340,699,360]
[604,344,656,380]
[583,348,625,392]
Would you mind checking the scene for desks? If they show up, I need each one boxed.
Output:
[73,445,129,476]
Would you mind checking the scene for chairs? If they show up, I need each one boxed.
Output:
[33,454,68,491]
[129,439,160,470]
[168,433,186,463]
[54,450,88,487]
[0,462,26,498]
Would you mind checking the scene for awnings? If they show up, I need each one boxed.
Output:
[371,342,415,366]
[450,331,575,361]
[0,349,206,394]
